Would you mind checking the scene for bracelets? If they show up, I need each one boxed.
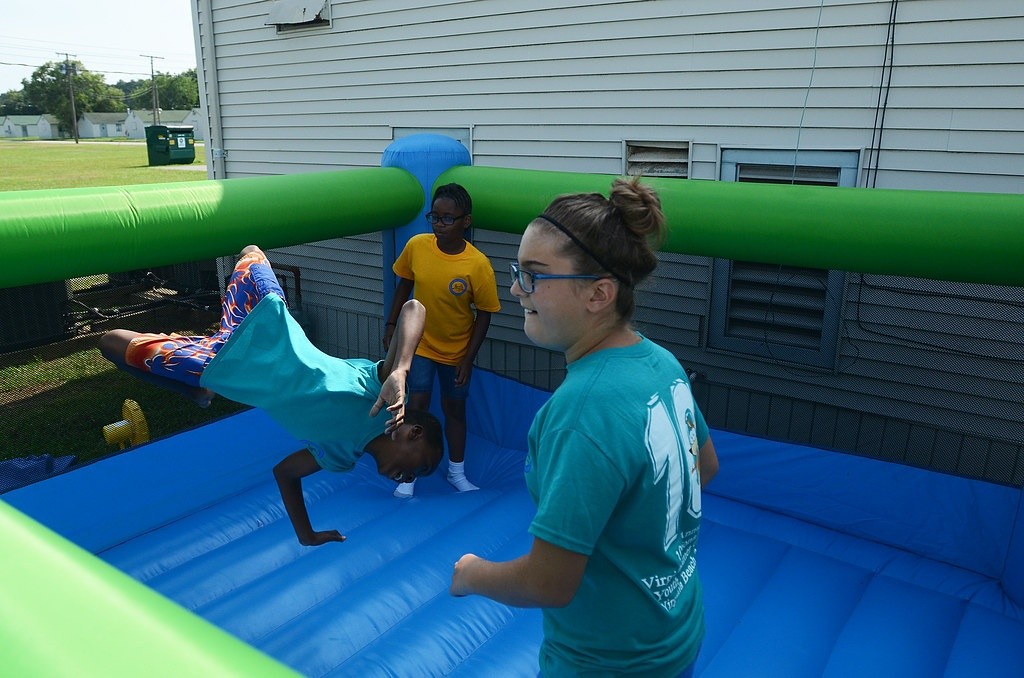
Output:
[385,323,395,326]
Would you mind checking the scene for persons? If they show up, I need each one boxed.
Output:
[451,182,721,678]
[383,183,501,499]
[98,245,445,547]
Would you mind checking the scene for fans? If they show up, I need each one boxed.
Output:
[102,398,150,451]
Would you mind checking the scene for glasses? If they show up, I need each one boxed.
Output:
[426,211,468,225]
[509,262,599,293]
